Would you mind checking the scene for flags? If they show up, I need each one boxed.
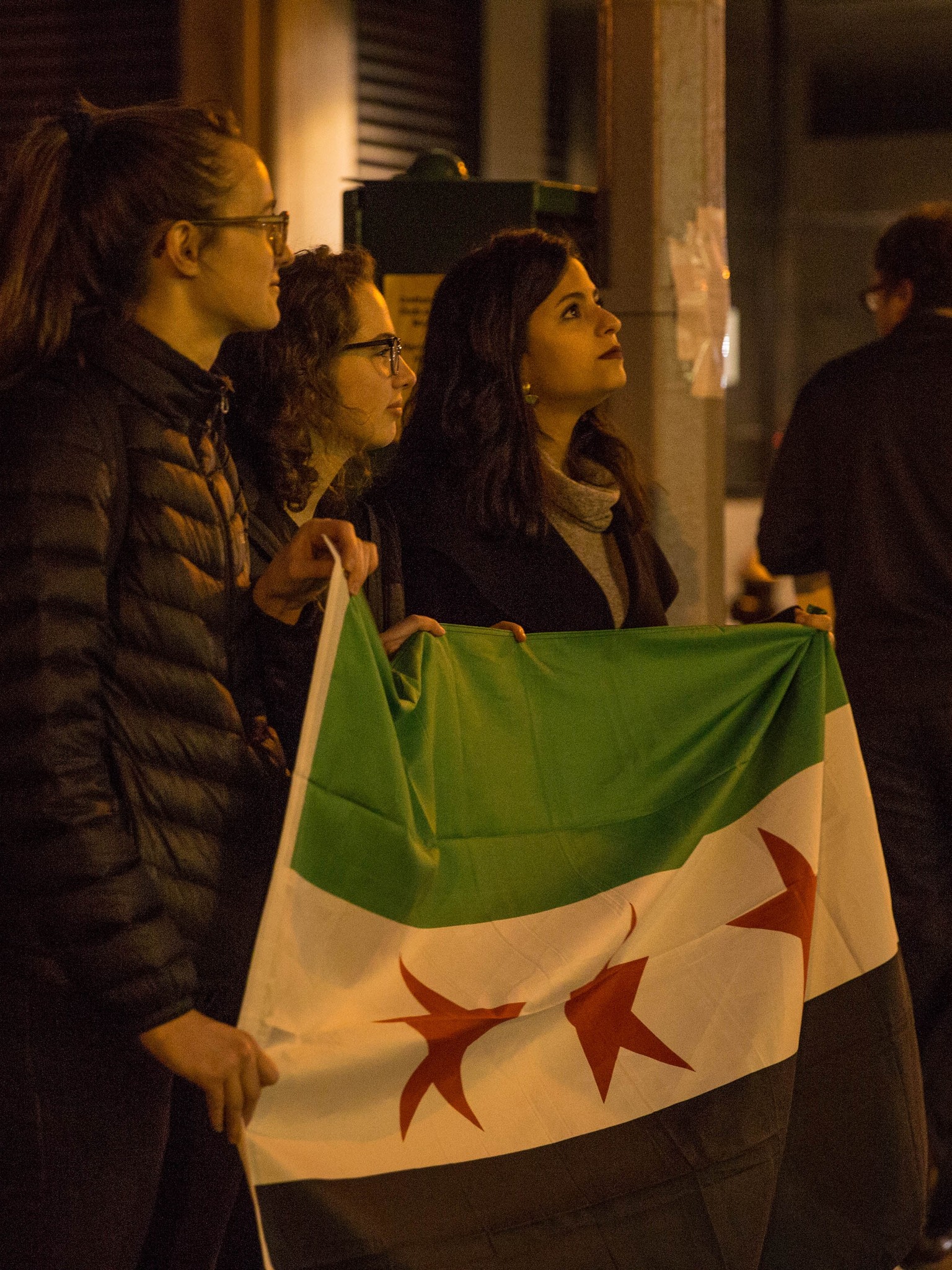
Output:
[229,533,934,1269]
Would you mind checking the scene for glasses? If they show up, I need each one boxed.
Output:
[335,336,403,375]
[149,210,288,260]
[856,277,896,314]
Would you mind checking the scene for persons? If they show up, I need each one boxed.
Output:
[1,102,948,1269]
